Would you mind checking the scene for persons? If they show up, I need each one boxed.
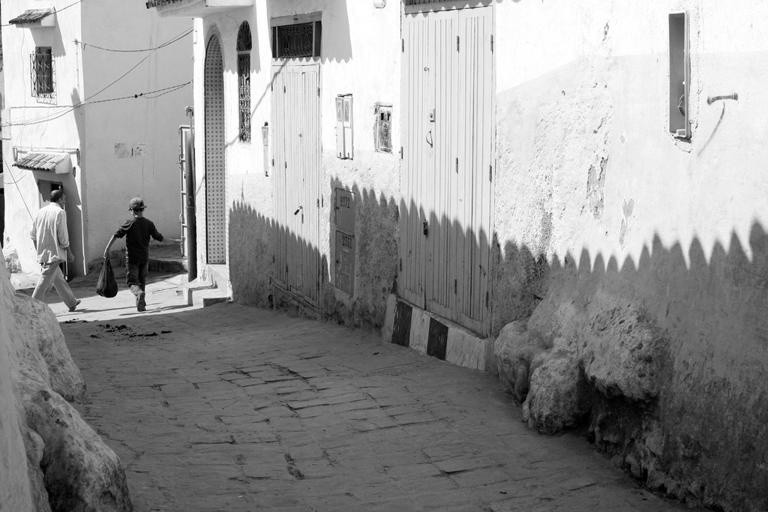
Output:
[103,197,163,311]
[28,189,81,313]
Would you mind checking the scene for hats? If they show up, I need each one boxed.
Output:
[128,197,147,210]
